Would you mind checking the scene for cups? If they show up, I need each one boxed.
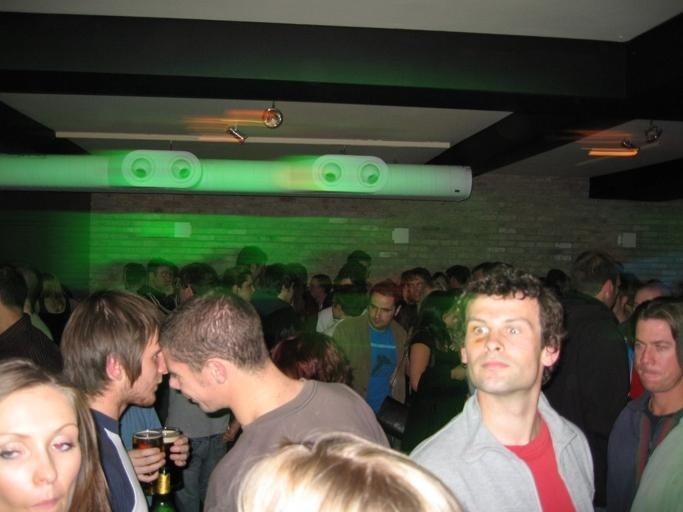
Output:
[131,424,183,495]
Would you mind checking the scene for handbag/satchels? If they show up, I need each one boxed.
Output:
[377,396,412,439]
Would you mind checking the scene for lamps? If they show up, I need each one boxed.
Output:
[619,120,665,151]
[224,100,285,145]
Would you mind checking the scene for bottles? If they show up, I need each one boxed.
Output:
[148,465,178,512]
